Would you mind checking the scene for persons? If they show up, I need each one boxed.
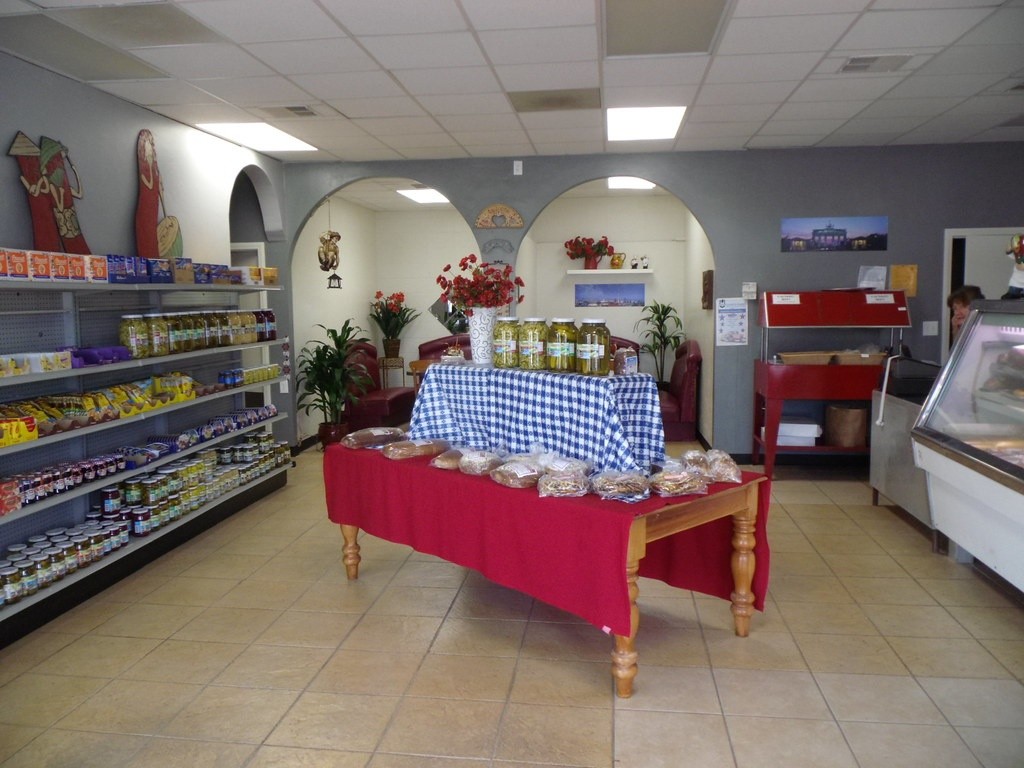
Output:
[946,285,988,354]
[1000,232,1024,301]
[318,231,341,271]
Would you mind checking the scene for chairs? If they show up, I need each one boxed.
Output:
[343,339,415,432]
[658,339,703,441]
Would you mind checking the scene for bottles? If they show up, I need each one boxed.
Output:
[642,255,648,269]
[631,256,637,269]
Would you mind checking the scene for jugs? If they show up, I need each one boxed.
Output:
[611,252,626,269]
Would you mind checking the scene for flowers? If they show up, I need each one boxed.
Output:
[370,291,422,338]
[565,236,614,260]
[437,254,526,317]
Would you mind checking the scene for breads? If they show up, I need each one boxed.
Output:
[340,426,740,497]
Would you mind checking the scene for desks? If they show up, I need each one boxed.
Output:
[323,442,772,699]
[377,357,404,388]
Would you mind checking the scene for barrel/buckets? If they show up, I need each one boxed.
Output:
[824,404,866,449]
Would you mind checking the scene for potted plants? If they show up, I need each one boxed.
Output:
[296,318,376,449]
[633,299,687,391]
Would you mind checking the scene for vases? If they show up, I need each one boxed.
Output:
[467,307,497,364]
[382,338,400,358]
[585,256,602,269]
[610,253,627,269]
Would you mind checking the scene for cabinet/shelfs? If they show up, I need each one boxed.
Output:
[0,277,296,649]
[753,358,881,477]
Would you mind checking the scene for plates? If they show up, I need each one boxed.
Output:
[823,287,877,290]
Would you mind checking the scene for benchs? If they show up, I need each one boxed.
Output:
[418,335,640,382]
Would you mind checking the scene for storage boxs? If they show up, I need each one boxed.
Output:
[777,416,820,446]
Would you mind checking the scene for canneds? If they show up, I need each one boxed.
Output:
[492,316,611,376]
[117,308,277,359]
[0,430,292,612]
